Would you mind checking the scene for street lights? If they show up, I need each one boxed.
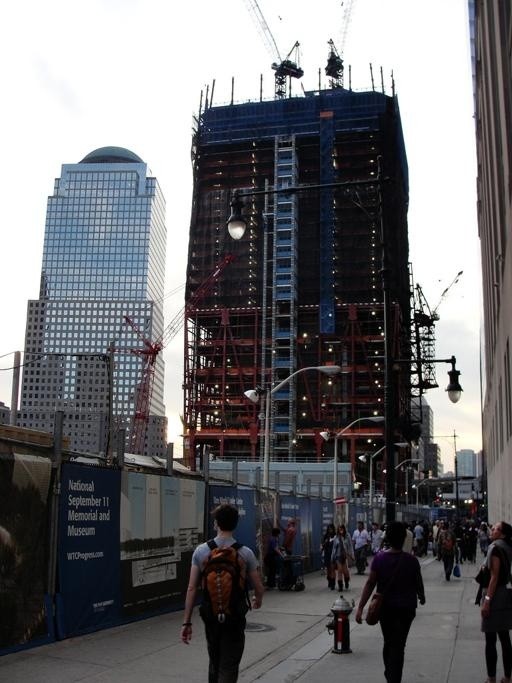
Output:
[411,476,439,507]
[243,364,341,495]
[358,441,409,506]
[225,155,463,406]
[382,458,424,503]
[319,416,385,498]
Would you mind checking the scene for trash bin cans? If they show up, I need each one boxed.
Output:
[278,555,309,591]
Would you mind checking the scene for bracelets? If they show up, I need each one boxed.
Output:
[484,594,493,601]
[182,621,193,626]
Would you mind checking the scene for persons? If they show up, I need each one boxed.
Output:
[331,522,356,592]
[355,521,425,682]
[179,503,266,683]
[321,522,336,590]
[474,519,511,683]
[274,513,298,555]
[368,515,495,582]
[265,527,284,591]
[351,519,372,575]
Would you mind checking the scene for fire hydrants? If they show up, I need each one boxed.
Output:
[325,594,356,653]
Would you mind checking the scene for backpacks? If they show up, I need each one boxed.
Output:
[196,538,250,624]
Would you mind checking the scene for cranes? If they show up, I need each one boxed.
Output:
[107,252,236,456]
[325,0,356,90]
[245,0,303,101]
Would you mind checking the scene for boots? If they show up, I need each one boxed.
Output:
[326,574,350,591]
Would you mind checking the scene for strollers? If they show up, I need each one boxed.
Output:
[276,554,308,592]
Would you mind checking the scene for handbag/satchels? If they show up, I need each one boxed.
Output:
[453,564,461,578]
[365,590,385,626]
